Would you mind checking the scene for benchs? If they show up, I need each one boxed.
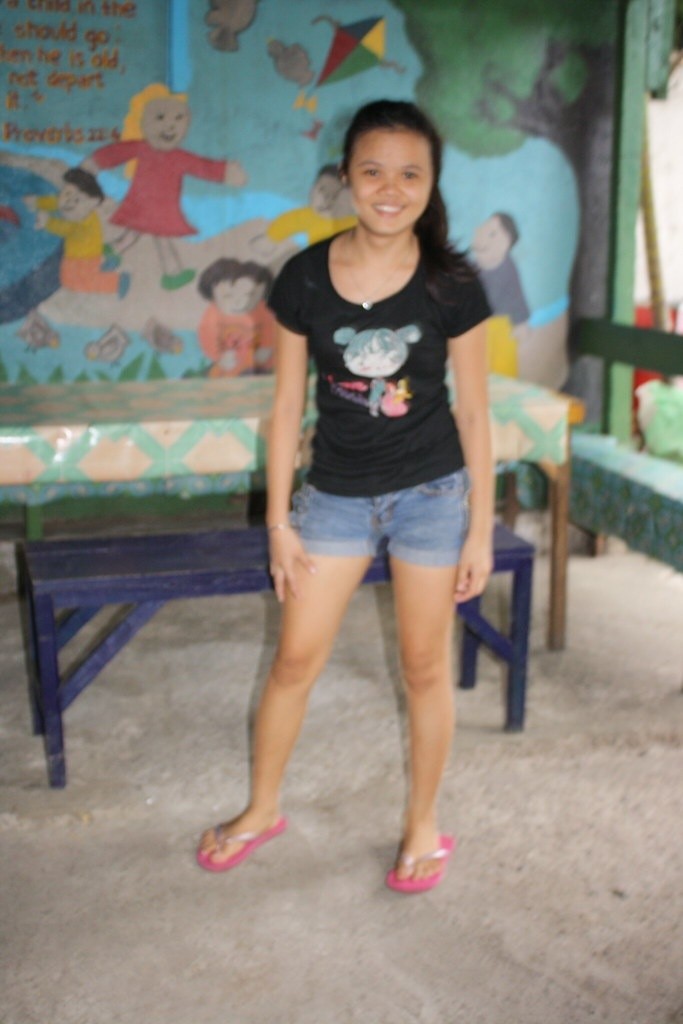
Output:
[16,524,535,788]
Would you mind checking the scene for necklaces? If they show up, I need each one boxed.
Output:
[340,231,411,310]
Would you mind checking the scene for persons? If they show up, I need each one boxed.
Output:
[199,98,494,891]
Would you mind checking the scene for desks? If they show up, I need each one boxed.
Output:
[0,374,584,650]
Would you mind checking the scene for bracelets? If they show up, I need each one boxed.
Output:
[266,525,291,531]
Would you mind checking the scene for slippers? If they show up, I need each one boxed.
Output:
[197,812,288,874]
[385,833,451,894]
